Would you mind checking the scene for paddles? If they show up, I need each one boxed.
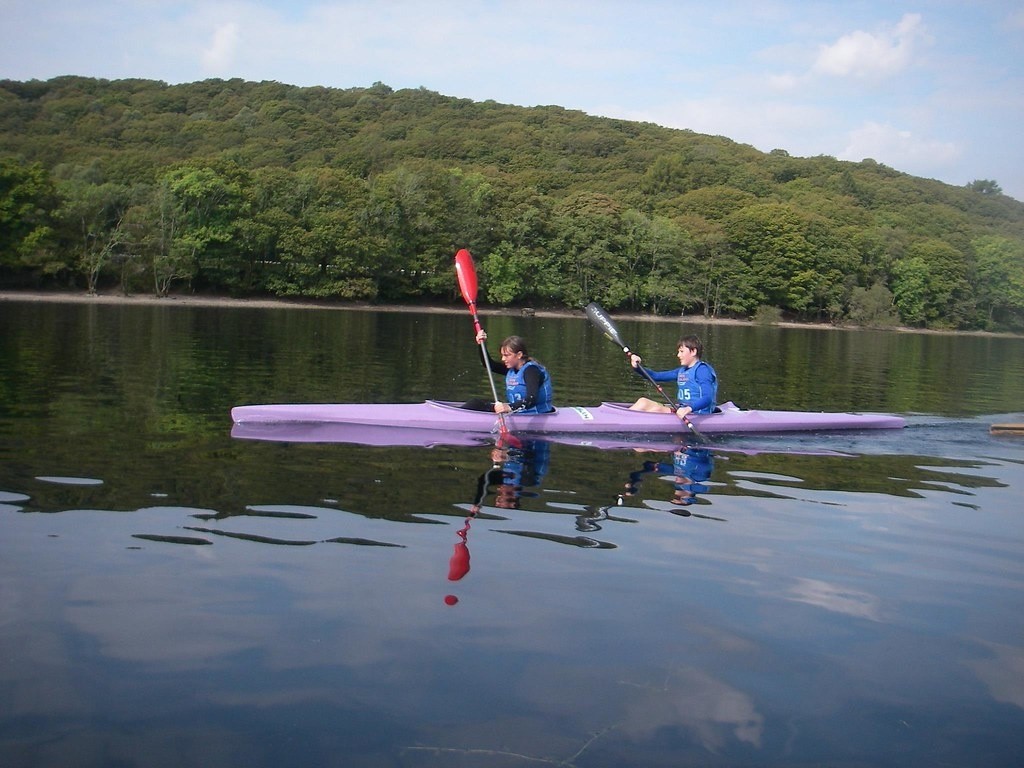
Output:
[585,301,718,447]
[455,249,522,451]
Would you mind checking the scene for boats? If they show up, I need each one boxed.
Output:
[232,400,908,434]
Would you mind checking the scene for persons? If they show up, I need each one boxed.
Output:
[459,329,553,412]
[626,333,718,414]
